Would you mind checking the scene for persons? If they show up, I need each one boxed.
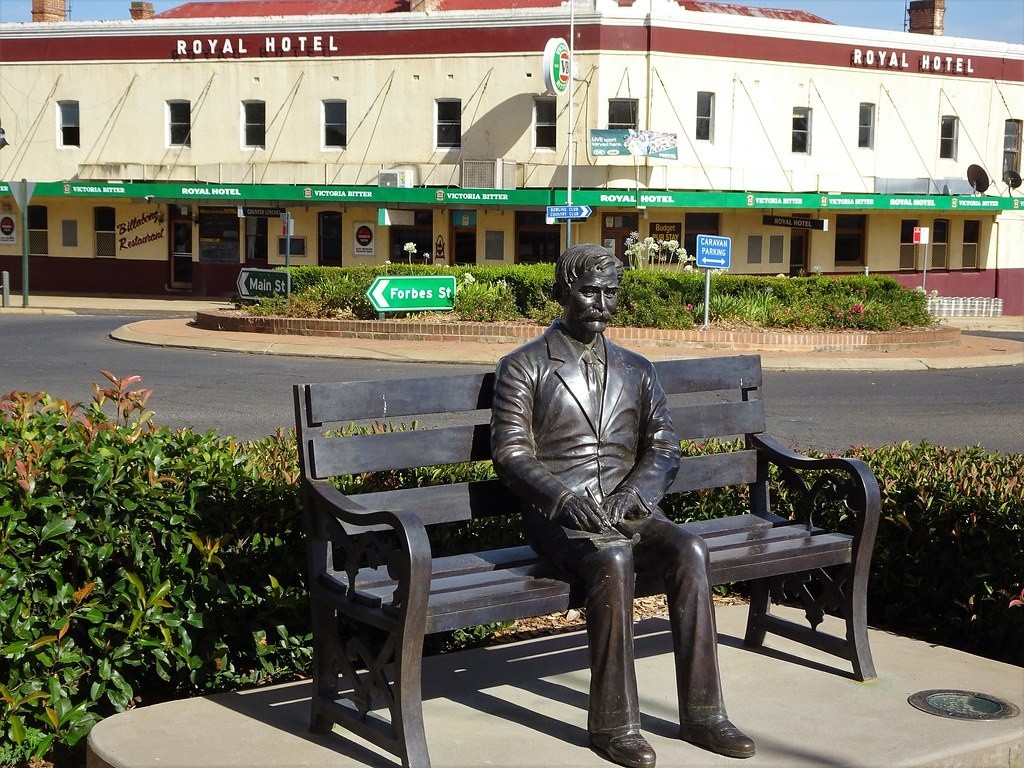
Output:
[490,243,756,768]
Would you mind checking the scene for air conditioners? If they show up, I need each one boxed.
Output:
[378,169,414,189]
[461,157,516,191]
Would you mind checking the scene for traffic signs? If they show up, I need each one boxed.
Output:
[547,204,593,221]
[695,233,733,269]
[233,266,291,303]
[364,275,459,313]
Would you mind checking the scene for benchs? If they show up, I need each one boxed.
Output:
[292,355,881,768]
